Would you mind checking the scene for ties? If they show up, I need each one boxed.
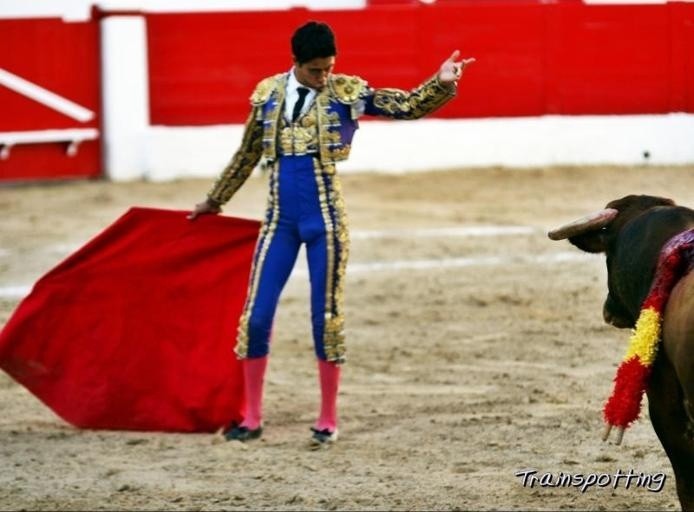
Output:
[291,88,310,122]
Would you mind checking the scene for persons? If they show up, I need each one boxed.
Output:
[184,19,475,453]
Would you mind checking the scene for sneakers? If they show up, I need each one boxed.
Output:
[307,427,336,451]
[221,419,260,441]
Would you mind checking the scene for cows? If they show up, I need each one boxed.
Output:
[548,194,694,512]
